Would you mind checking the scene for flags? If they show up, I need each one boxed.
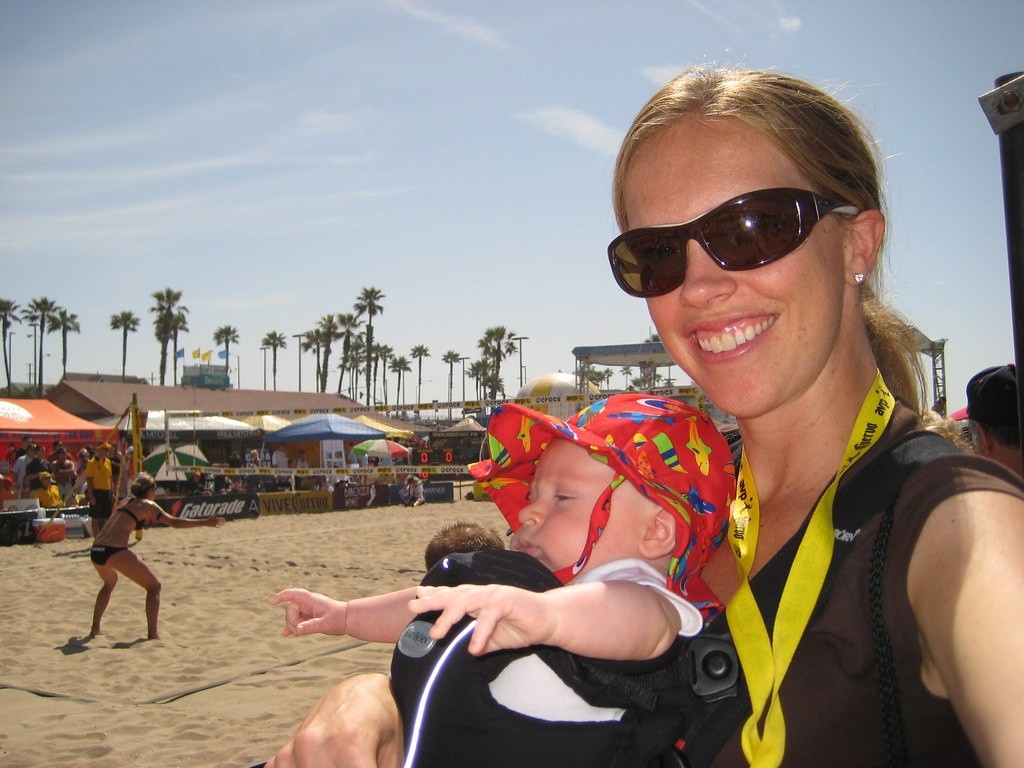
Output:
[177,348,226,362]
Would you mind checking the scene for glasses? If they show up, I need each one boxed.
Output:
[607,187,859,298]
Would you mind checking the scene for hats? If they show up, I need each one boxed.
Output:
[96,441,112,449]
[949,363,1019,427]
[467,394,737,631]
[39,471,51,479]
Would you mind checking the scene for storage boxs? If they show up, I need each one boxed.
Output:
[32,517,67,542]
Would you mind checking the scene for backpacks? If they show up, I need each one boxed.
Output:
[14,518,34,544]
[0,519,16,545]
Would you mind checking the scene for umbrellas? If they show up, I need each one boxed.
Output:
[142,443,209,493]
[352,415,413,440]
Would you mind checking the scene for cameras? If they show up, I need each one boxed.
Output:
[408,477,414,484]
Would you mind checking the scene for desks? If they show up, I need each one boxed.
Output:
[0,505,90,546]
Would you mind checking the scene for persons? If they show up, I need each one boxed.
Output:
[271,393,737,768]
[966,364,1024,478]
[404,476,425,507]
[374,437,437,466]
[273,443,308,491]
[0,435,145,539]
[209,450,261,495]
[347,442,368,485]
[724,215,777,268]
[641,235,682,293]
[267,67,1024,768]
[91,475,226,640]
[184,473,207,496]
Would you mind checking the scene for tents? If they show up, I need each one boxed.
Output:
[242,416,289,432]
[0,398,128,494]
[264,413,396,482]
[353,439,409,468]
[91,415,265,468]
[429,416,488,462]
